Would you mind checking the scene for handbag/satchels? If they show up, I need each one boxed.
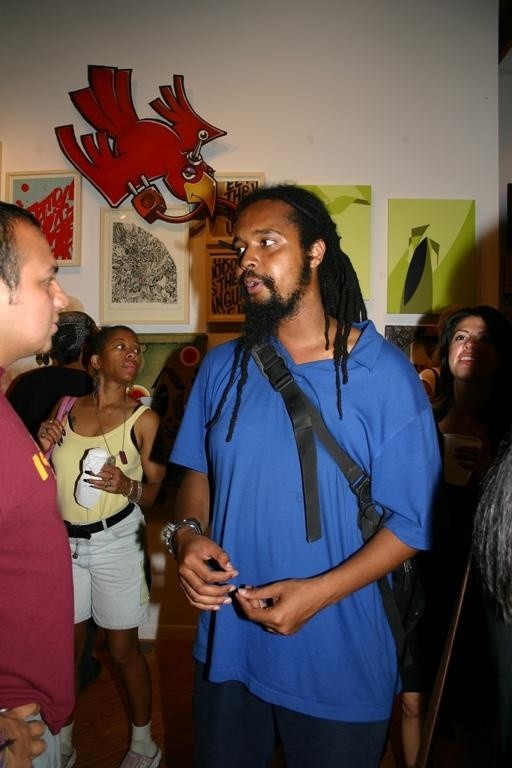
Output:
[368,502,441,664]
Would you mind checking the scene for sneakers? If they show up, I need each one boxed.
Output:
[120,740,162,768]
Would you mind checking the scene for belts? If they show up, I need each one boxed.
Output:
[61,501,136,541]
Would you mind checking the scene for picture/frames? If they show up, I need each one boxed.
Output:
[102,203,190,325]
[6,169,82,266]
[202,168,269,248]
[204,245,249,326]
